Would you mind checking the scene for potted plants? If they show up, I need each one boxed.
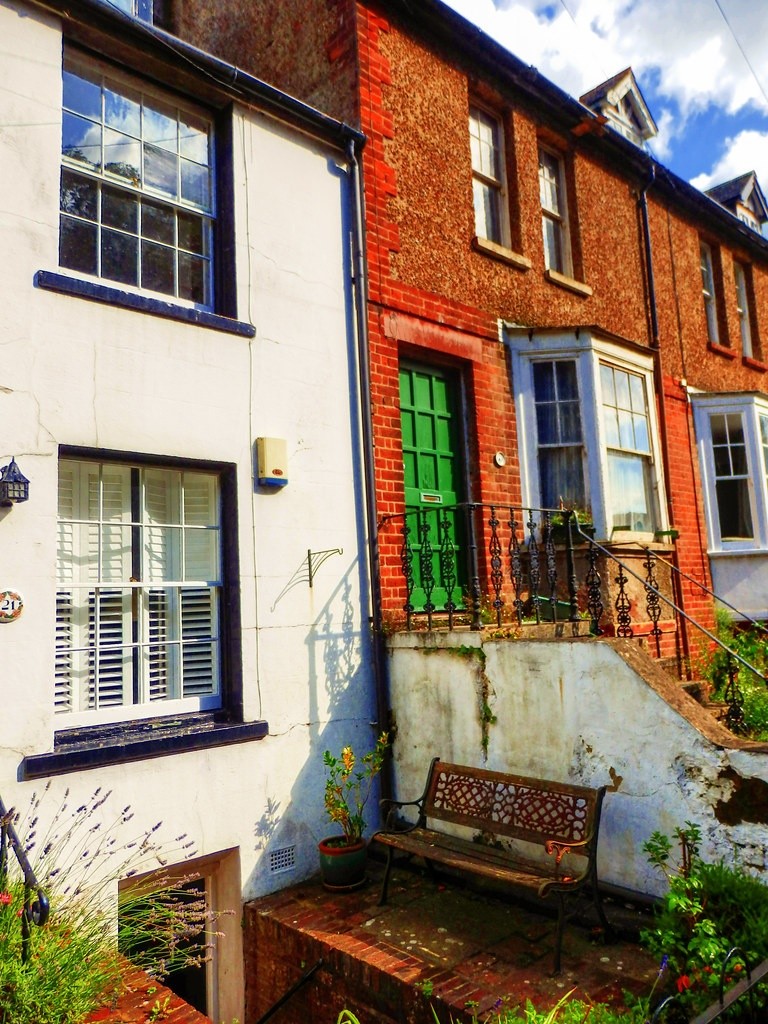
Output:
[545,507,592,543]
[318,731,391,890]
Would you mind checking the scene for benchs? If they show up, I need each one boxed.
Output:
[366,757,613,975]
